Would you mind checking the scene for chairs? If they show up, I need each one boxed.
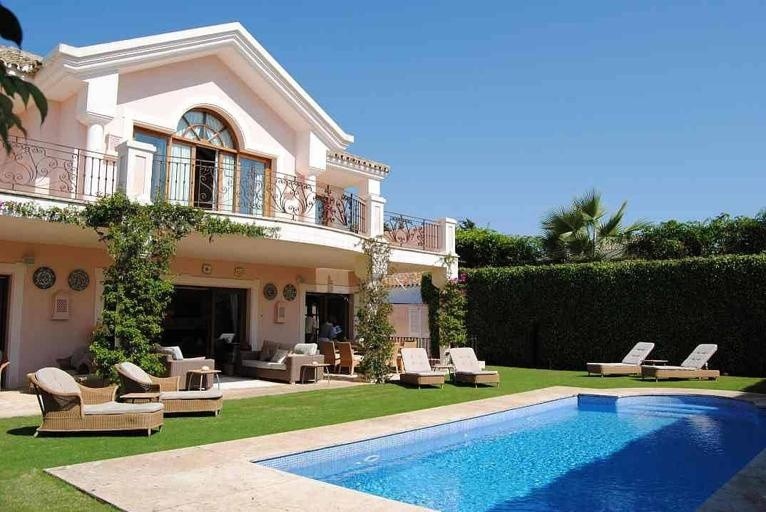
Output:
[26,367,165,441]
[586,342,656,378]
[159,346,216,388]
[447,347,502,389]
[404,341,417,348]
[400,347,445,390]
[641,341,722,383]
[385,342,399,374]
[111,360,226,417]
[336,342,364,375]
[319,341,341,374]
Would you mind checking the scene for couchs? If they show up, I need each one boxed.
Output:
[237,339,326,385]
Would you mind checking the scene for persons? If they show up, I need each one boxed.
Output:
[305,311,316,341]
[320,315,333,338]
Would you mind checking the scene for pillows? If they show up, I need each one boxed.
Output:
[270,349,288,363]
[294,343,317,354]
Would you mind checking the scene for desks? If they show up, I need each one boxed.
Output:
[120,392,162,402]
[186,368,222,392]
[431,364,455,380]
[641,359,669,366]
[300,363,332,384]
